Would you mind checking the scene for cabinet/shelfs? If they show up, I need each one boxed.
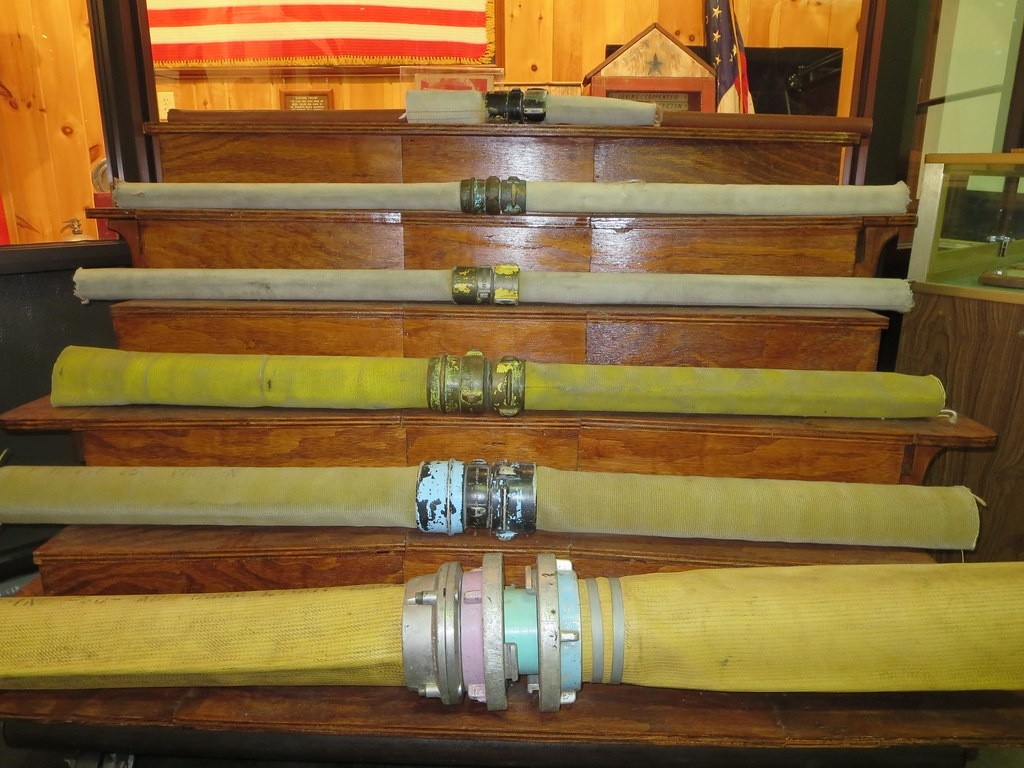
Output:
[899,153,1023,562]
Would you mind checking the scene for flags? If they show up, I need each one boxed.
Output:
[703,0,755,114]
